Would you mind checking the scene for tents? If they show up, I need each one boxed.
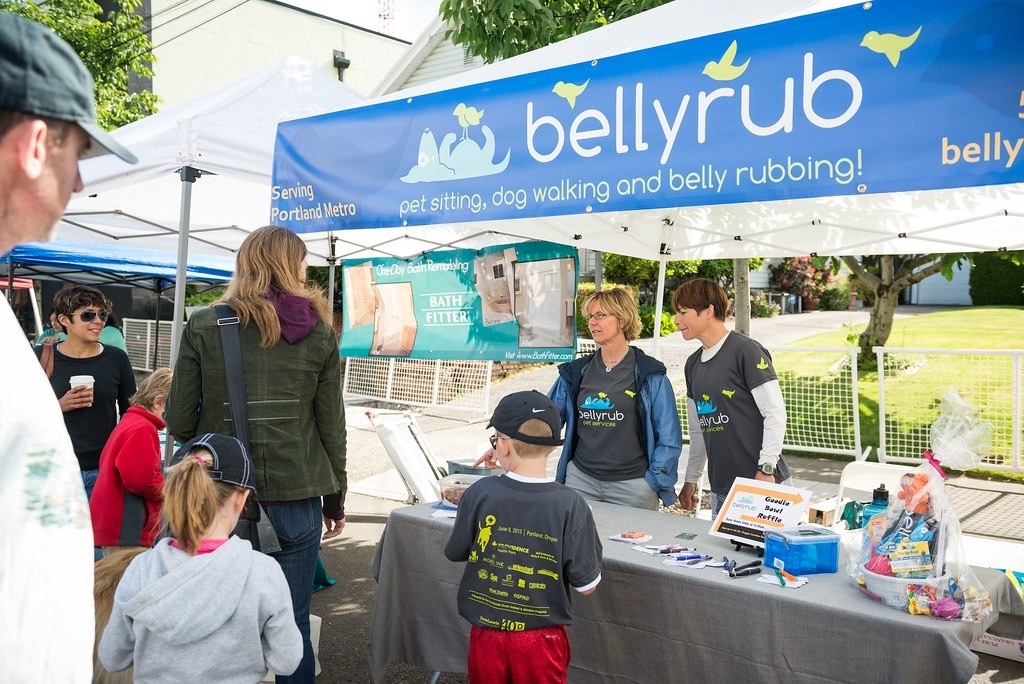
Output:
[0,234,240,371]
[267,2,1024,360]
[46,47,366,433]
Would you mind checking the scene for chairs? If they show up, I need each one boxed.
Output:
[369,401,454,509]
[676,438,722,523]
[839,449,942,546]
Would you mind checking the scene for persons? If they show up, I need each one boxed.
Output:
[96,431,303,684]
[160,224,348,684]
[89,365,174,558]
[31,286,138,561]
[444,389,604,684]
[672,280,793,520]
[0,9,139,684]
[470,288,682,513]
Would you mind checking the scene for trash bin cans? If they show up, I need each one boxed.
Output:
[447,456,506,476]
[849,292,857,307]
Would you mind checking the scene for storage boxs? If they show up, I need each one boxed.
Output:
[760,520,840,577]
[808,497,840,526]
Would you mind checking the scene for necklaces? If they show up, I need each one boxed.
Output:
[602,344,629,372]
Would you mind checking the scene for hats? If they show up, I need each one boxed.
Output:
[487,390,566,447]
[0,8,138,163]
[169,432,261,524]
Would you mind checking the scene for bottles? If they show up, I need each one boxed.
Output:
[861,483,891,530]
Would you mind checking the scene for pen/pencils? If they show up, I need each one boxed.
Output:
[732,560,762,571]
[729,560,736,572]
[729,569,763,577]
[687,557,713,565]
[722,556,729,570]
[673,555,708,560]
[775,568,798,586]
[653,547,696,554]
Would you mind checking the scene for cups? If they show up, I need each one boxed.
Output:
[69,375,95,408]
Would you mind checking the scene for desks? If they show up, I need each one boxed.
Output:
[390,501,1006,684]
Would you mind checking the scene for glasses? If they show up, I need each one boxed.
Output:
[490,434,513,450]
[584,311,619,323]
[68,309,109,323]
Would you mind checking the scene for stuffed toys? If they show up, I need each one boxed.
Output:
[866,469,940,577]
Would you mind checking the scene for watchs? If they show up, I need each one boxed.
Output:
[756,464,778,476]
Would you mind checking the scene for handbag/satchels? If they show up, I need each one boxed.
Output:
[229,498,282,555]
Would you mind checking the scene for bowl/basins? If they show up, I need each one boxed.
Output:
[438,474,486,508]
[859,559,952,609]
